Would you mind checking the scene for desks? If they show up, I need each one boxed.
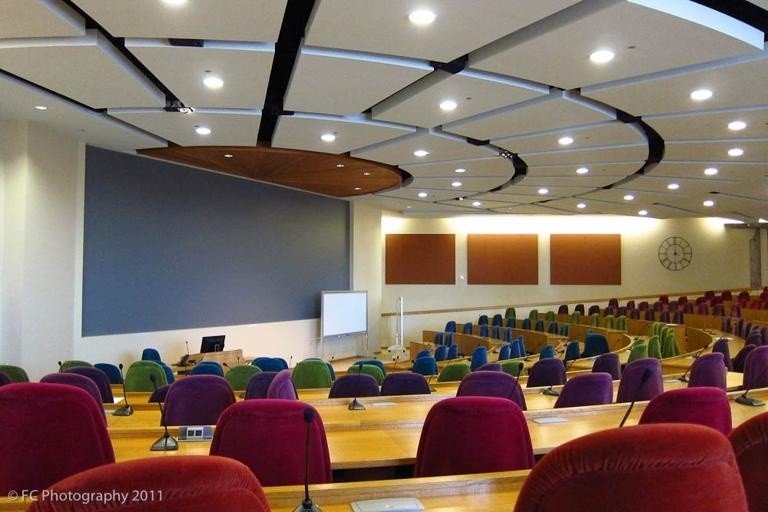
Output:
[411,290,764,381]
[0,469,536,508]
[105,387,764,471]
[184,349,243,368]
[103,381,463,402]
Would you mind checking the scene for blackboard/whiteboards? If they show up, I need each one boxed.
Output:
[321,290,367,337]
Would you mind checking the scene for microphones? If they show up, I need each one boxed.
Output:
[57,361,63,372]
[289,356,291,369]
[112,364,133,416]
[236,357,239,365]
[148,374,179,452]
[426,366,437,392]
[348,362,365,410]
[392,356,398,373]
[178,341,189,367]
[188,360,198,365]
[618,367,652,428]
[292,407,323,512]
[611,339,621,351]
[222,363,231,369]
[329,356,333,366]
[678,344,707,383]
[507,363,523,399]
[543,352,593,396]
[735,364,768,407]
[524,343,541,361]
[558,349,565,360]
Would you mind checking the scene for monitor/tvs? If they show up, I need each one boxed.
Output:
[200,335,226,353]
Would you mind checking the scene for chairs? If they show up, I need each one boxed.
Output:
[208,400,332,490]
[616,358,664,404]
[409,299,626,388]
[124,349,175,394]
[225,364,264,392]
[190,360,224,376]
[159,377,236,427]
[554,373,616,409]
[411,400,536,477]
[250,355,289,373]
[27,456,273,512]
[639,387,733,435]
[743,343,767,388]
[731,412,767,512]
[347,358,386,387]
[625,283,767,362]
[292,358,335,390]
[266,369,298,402]
[687,352,729,392]
[64,359,124,426]
[457,371,528,412]
[327,375,379,400]
[513,424,750,512]
[381,372,430,397]
[0,383,116,500]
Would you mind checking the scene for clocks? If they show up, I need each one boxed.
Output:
[651,231,699,279]
